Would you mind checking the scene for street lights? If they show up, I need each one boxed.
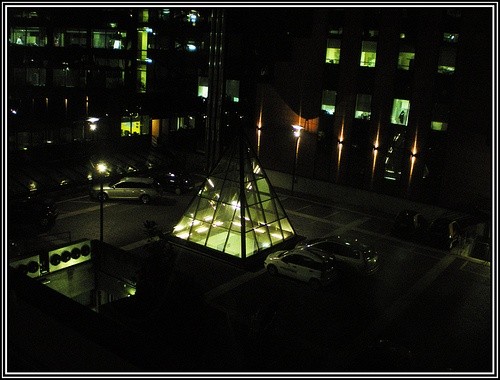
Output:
[86,117,100,164]
[291,125,304,192]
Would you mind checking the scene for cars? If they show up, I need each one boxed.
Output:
[7,192,59,234]
[391,210,462,249]
[263,248,337,290]
[144,168,193,194]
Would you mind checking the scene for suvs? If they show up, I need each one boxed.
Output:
[90,177,163,204]
[295,236,380,289]
